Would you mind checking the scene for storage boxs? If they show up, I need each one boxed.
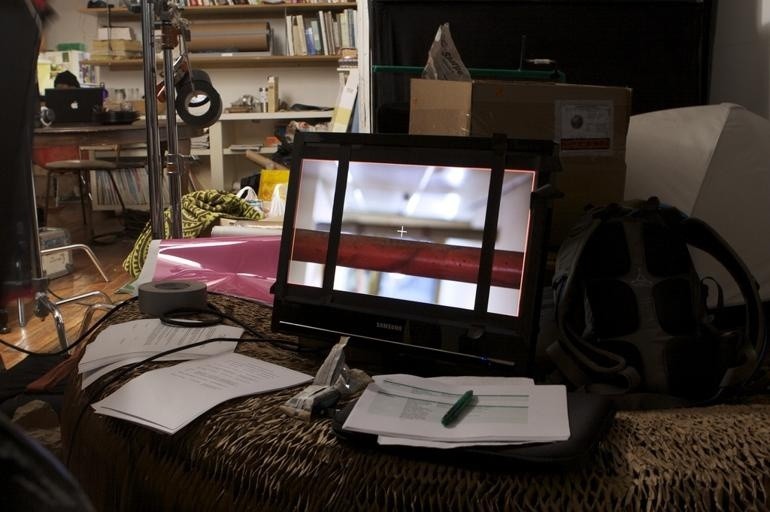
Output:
[402,78,631,247]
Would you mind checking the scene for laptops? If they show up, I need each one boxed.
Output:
[45,89,103,126]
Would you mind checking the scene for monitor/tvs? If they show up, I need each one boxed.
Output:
[271,132,559,377]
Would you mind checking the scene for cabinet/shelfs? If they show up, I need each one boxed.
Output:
[76,0,356,63]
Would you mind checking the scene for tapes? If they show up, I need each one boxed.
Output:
[138,281,207,315]
[176,70,222,128]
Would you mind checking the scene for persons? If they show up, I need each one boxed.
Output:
[54,70,80,89]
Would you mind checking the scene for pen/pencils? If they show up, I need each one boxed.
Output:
[441,391,473,426]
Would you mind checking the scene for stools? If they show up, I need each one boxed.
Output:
[42,159,128,226]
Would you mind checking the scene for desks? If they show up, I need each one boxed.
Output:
[29,110,338,210]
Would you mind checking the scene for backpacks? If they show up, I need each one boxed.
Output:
[551,192,766,407]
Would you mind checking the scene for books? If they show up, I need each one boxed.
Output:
[229,136,290,153]
[91,0,360,56]
[224,72,278,113]
[93,167,205,210]
[190,134,210,149]
[81,64,140,102]
